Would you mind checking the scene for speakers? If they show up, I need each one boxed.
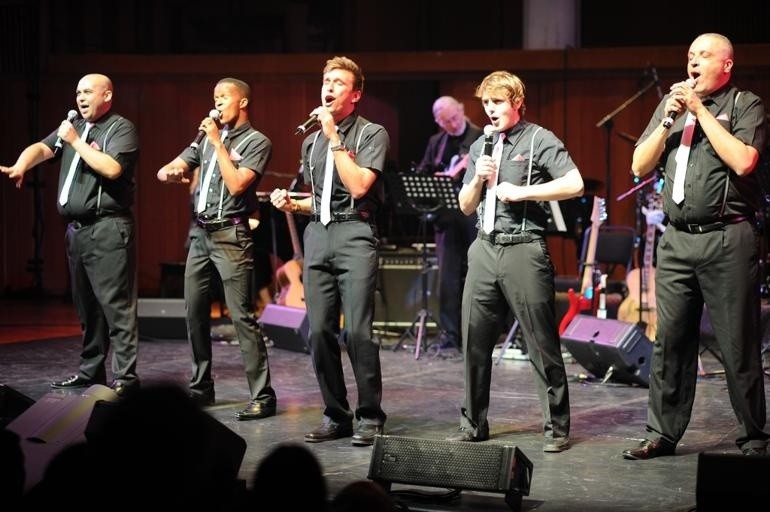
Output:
[136,299,190,340]
[256,303,314,356]
[369,434,533,497]
[563,314,653,384]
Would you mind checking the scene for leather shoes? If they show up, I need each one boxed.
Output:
[188,390,217,406]
[542,437,571,453]
[49,372,107,389]
[109,380,141,396]
[429,341,460,355]
[743,447,767,456]
[303,420,353,443]
[350,422,384,446]
[620,436,678,460]
[444,431,488,443]
[232,399,278,420]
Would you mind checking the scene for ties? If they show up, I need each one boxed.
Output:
[481,132,509,239]
[195,128,230,213]
[671,109,702,205]
[56,120,97,208]
[319,124,341,227]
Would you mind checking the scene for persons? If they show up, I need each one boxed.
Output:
[621,31,770,457]
[155,77,280,420]
[422,96,484,350]
[0,383,409,511]
[270,56,391,445]
[444,70,586,454]
[0,73,139,397]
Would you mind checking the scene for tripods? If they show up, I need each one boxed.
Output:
[390,247,462,359]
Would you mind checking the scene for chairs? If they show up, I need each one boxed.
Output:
[493,223,637,365]
[159,261,186,297]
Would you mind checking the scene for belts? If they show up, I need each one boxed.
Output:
[62,210,134,227]
[474,229,545,246]
[666,212,752,235]
[191,211,250,233]
[310,211,364,226]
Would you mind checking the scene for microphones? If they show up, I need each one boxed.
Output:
[294,115,322,135]
[190,110,220,148]
[663,78,696,130]
[483,125,496,157]
[53,109,77,152]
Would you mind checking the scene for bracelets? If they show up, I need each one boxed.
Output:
[293,197,299,215]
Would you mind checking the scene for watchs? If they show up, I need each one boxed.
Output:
[328,141,347,154]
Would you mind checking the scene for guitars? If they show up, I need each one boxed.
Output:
[617,194,663,341]
[275,205,309,309]
[559,197,608,333]
